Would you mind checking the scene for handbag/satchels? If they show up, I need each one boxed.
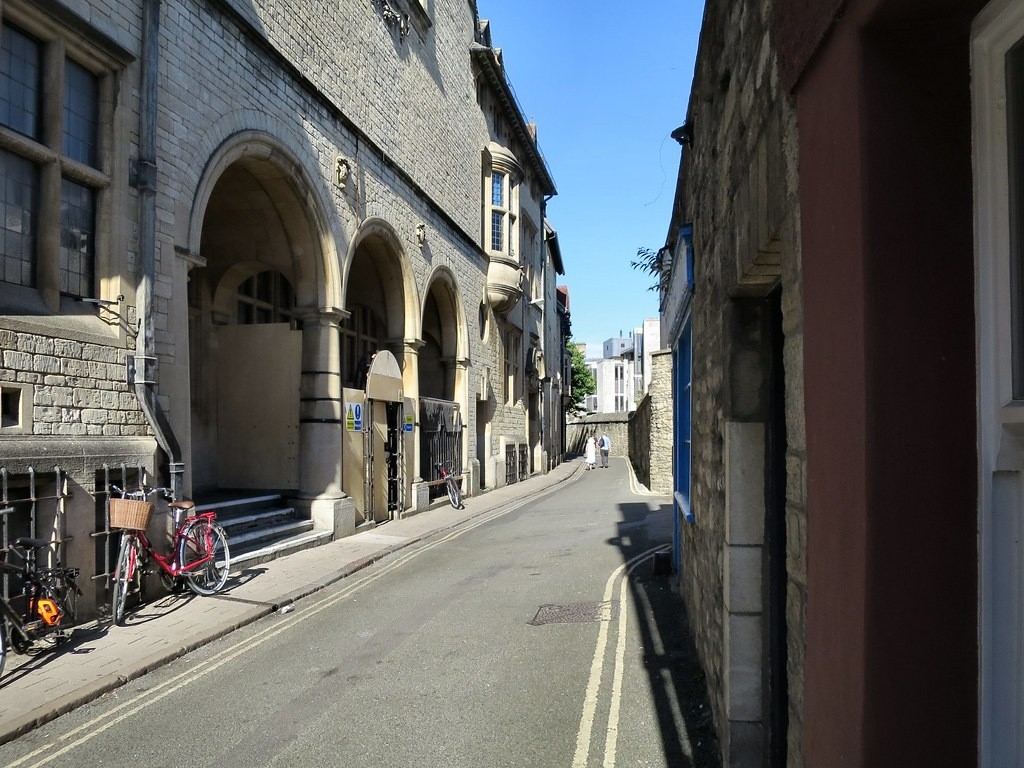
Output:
[599,437,604,448]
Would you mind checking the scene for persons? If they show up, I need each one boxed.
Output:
[586,433,598,470]
[598,433,611,468]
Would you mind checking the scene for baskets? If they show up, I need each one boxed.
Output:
[110,498,154,531]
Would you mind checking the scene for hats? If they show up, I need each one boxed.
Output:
[588,438,595,443]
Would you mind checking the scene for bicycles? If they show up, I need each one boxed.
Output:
[435,460,462,510]
[0,506,84,685]
[106,481,231,626]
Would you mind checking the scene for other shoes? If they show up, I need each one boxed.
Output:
[601,466,605,468]
[592,466,595,469]
[605,465,608,468]
[586,468,590,470]
